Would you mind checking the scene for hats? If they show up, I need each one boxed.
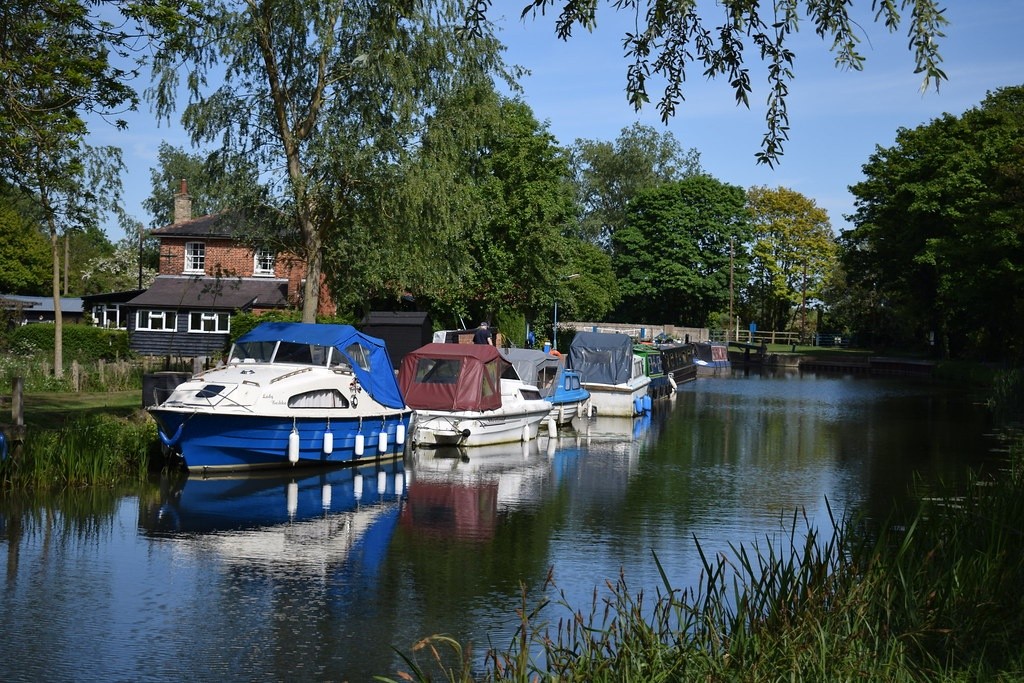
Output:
[480,322,487,326]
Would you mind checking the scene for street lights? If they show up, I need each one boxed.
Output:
[553,273,580,350]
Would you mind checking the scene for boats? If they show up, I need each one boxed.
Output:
[499,347,592,426]
[630,327,733,401]
[396,342,555,448]
[144,320,415,475]
[568,327,653,419]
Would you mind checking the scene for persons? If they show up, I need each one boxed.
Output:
[472,322,493,345]
